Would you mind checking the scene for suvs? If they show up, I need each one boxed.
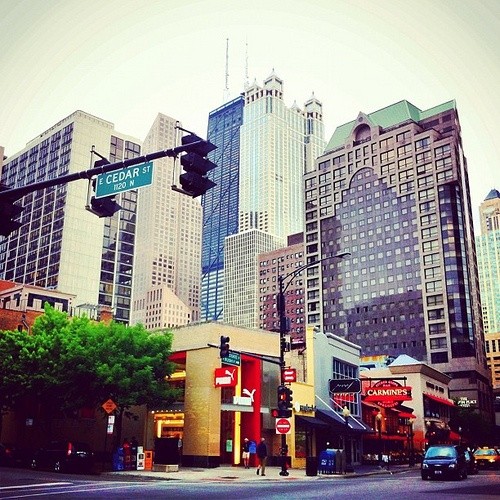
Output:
[421,444,467,480]
[31,440,94,473]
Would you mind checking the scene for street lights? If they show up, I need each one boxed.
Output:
[276,253,352,476]
[376,412,384,469]
[342,406,354,472]
[408,417,415,468]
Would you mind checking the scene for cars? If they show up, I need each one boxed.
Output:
[464,447,500,474]
[0,443,10,466]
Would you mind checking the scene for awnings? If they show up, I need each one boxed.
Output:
[295,408,374,434]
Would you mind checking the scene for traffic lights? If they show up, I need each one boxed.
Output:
[271,385,293,418]
[220,336,229,358]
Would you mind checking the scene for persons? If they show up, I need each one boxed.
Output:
[242,438,252,469]
[256,438,268,476]
[110,436,139,458]
